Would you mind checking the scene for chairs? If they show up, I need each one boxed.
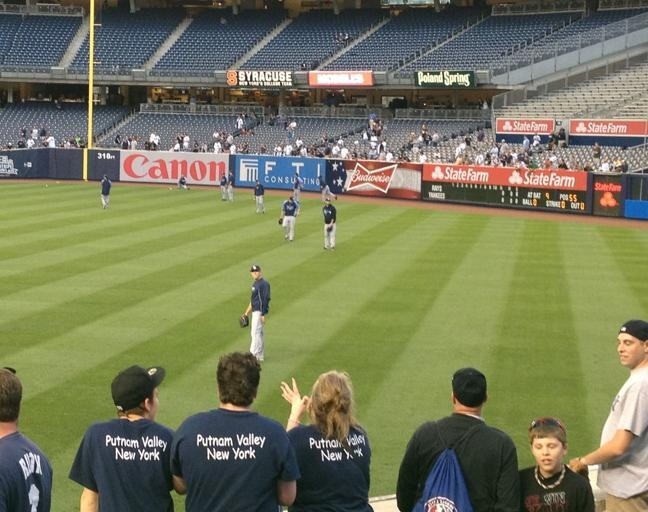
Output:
[0,10,648,171]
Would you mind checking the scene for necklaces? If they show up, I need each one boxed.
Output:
[534,464,565,490]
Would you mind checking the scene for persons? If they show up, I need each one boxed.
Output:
[519,417,595,512]
[281,173,303,241]
[169,352,301,512]
[102,175,112,208]
[254,179,265,214]
[569,320,648,512]
[114,129,162,151]
[0,368,53,512]
[241,265,271,361]
[454,127,629,173]
[362,114,440,162]
[221,171,234,202]
[7,125,85,148]
[396,368,521,512]
[279,370,374,512]
[213,113,266,154]
[318,175,337,249]
[68,365,176,512]
[274,118,357,161]
[169,132,207,153]
[178,175,187,188]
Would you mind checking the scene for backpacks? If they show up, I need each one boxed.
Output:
[410,421,482,511]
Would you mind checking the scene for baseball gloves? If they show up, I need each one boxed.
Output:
[279,218,283,223]
[327,226,332,231]
[240,316,249,328]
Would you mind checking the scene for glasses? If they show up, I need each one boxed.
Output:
[529,417,565,433]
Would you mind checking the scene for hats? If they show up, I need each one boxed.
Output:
[111,365,166,412]
[250,265,260,272]
[453,367,486,405]
[620,319,648,342]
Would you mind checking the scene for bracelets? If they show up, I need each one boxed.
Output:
[579,456,587,466]
[288,418,301,425]
